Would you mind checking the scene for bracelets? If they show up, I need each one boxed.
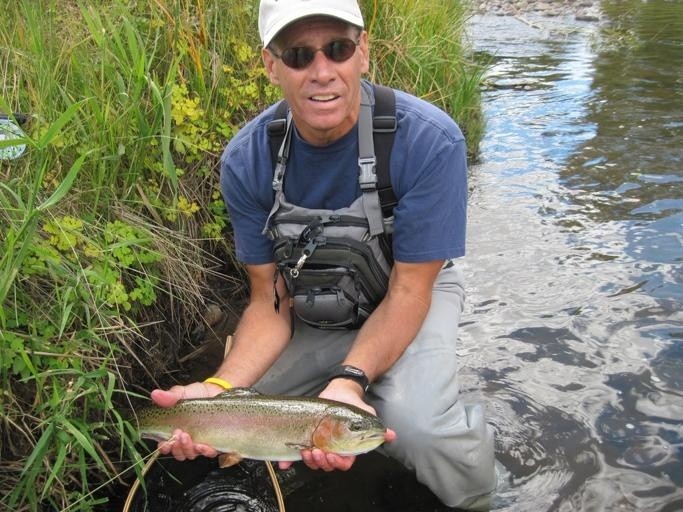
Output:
[203,378,232,389]
[328,365,371,396]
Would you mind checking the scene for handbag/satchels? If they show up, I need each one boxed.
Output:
[263,192,395,333]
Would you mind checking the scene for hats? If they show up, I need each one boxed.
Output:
[258,0,364,50]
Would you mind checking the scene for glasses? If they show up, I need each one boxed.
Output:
[266,39,360,70]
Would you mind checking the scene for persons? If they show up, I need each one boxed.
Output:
[150,4,496,512]
